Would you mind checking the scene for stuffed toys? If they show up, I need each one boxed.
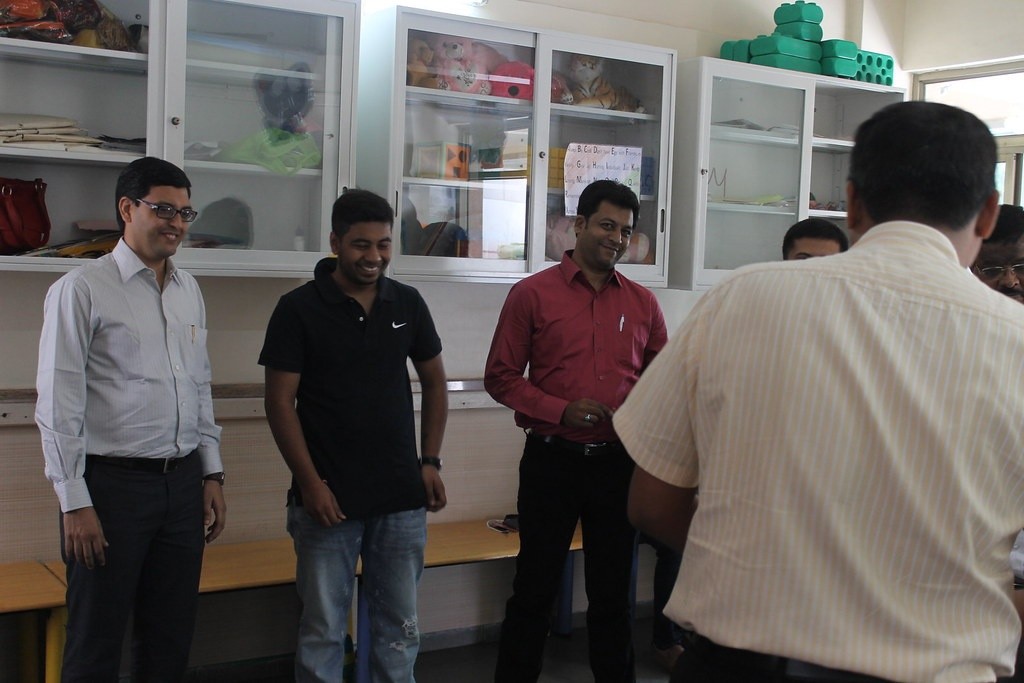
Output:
[426,32,491,95]
[407,36,440,89]
[564,51,644,113]
[474,43,566,104]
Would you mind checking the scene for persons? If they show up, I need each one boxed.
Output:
[257,189,448,683]
[973,204,1024,683]
[643,217,850,672]
[613,100,1024,683]
[483,180,668,683]
[34,157,227,683]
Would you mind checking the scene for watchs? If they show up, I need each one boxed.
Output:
[204,471,225,485]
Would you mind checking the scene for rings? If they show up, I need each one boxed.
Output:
[585,414,591,421]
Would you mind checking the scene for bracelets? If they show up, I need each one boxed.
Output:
[421,456,443,470]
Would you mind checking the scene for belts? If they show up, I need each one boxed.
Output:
[86,448,198,474]
[542,435,621,459]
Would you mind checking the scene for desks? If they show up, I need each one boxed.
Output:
[0,521,586,683]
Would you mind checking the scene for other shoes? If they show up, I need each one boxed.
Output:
[652,643,685,674]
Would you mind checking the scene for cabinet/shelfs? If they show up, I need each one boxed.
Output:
[0,0,906,292]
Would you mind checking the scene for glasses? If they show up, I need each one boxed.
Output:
[124,192,198,222]
[975,264,1024,280]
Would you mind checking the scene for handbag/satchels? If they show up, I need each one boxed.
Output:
[0,178,51,255]
